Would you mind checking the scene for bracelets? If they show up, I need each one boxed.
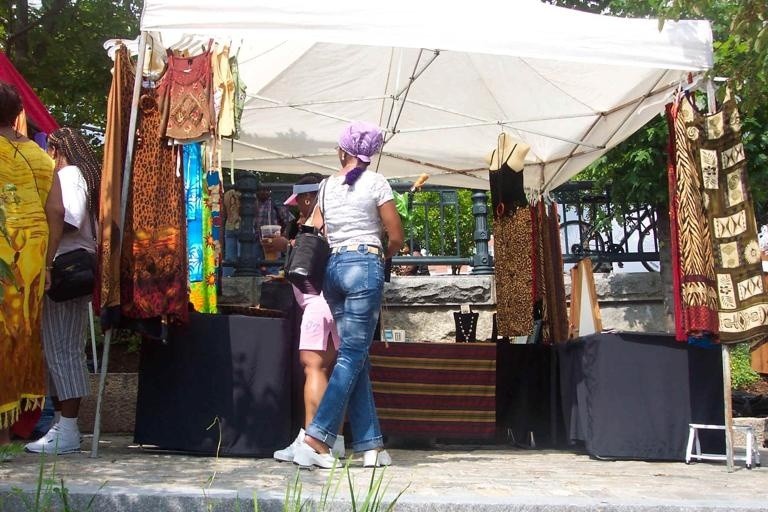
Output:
[46,266,54,271]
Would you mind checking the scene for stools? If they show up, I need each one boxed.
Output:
[684,422,761,469]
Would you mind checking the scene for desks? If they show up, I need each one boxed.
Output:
[357,336,500,451]
[493,339,562,445]
[135,310,295,457]
[562,331,692,462]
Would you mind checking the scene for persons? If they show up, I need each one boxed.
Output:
[273,176,345,461]
[26,128,102,455]
[401,239,430,275]
[292,122,393,466]
[486,134,534,344]
[1,83,66,462]
[224,183,306,277]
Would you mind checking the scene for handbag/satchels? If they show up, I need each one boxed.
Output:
[283,200,331,297]
[46,209,102,307]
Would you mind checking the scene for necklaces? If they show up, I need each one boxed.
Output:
[459,316,474,342]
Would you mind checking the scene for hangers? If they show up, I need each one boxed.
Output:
[635,70,741,116]
[103,30,244,63]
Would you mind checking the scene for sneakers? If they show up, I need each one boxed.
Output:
[364,446,393,467]
[333,433,345,459]
[297,440,342,468]
[39,409,61,432]
[275,426,309,462]
[26,418,83,454]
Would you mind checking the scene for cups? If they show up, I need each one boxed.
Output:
[261,225,281,260]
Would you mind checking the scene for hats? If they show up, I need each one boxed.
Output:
[283,184,318,205]
